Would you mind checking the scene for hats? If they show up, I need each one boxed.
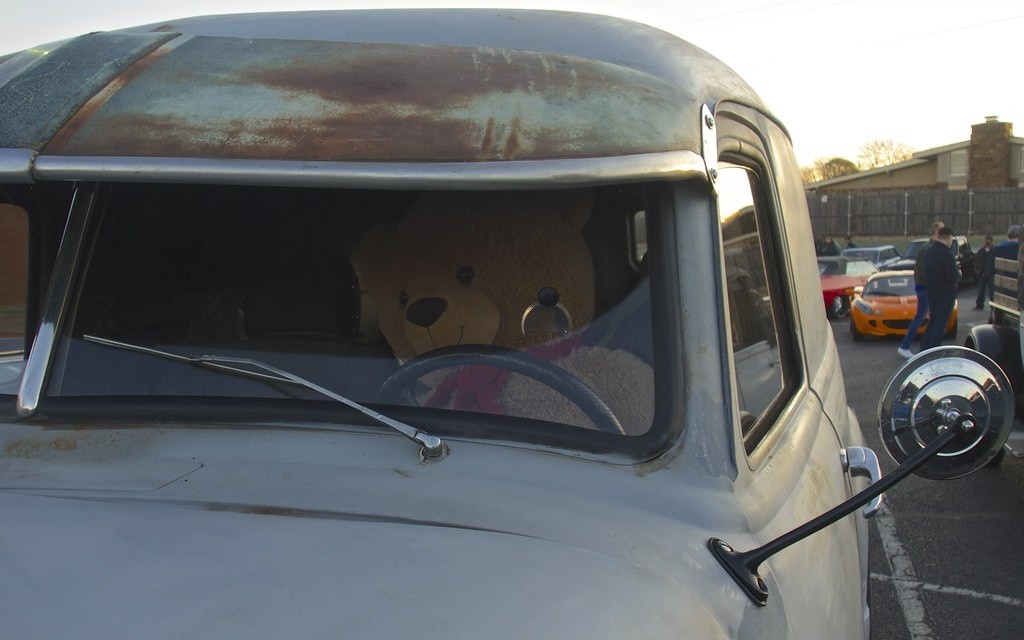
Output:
[938,226,956,236]
[1008,225,1021,235]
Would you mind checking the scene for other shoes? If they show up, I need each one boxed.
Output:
[976,304,984,309]
[898,347,914,358]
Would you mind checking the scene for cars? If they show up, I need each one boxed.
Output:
[0,8,1017,640]
[813,234,975,322]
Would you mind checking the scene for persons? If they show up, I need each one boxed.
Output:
[920,226,963,351]
[843,235,857,256]
[871,251,887,263]
[898,222,945,359]
[828,237,841,256]
[988,225,1021,323]
[1017,225,1024,312]
[968,252,978,287]
[976,235,995,309]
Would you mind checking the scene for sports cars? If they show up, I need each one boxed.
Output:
[850,269,960,342]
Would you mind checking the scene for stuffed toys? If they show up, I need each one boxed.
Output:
[358,186,655,435]
